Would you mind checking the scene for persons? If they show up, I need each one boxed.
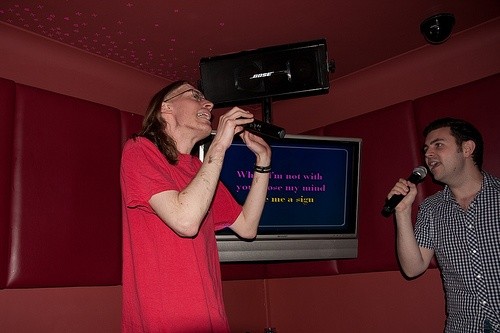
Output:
[120,80,271,333]
[387,117,500,333]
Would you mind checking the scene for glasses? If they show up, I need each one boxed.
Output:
[163,88,205,103]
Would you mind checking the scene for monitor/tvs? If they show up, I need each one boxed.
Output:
[199,129,363,262]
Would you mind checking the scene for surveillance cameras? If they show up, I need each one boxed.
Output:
[420,13,457,44]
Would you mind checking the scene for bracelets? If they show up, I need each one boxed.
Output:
[254,163,272,173]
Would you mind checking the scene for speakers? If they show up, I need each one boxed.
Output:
[198,39,330,108]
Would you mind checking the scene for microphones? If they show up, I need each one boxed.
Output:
[236,117,286,139]
[381,166,427,218]
